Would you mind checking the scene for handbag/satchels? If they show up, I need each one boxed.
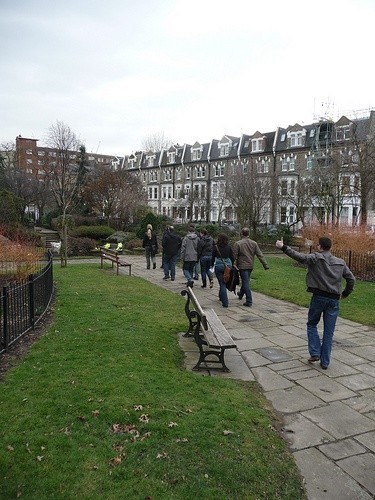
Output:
[223,267,231,283]
[224,267,237,291]
[152,245,158,254]
[233,266,240,286]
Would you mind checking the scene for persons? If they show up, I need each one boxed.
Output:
[181,228,216,288]
[142,224,158,269]
[232,228,269,306]
[160,225,182,280]
[276,236,355,370]
[210,233,240,307]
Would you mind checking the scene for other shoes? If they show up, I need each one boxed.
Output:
[237,291,242,300]
[193,277,198,280]
[210,281,214,288]
[222,304,228,307]
[243,303,251,307]
[201,286,206,288]
[147,268,150,269]
[153,262,156,269]
[171,278,174,281]
[187,281,194,288]
[219,298,222,301]
[320,362,327,370]
[308,356,319,361]
[163,276,170,279]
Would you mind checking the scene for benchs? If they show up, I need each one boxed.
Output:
[180,286,236,372]
[99,246,132,276]
